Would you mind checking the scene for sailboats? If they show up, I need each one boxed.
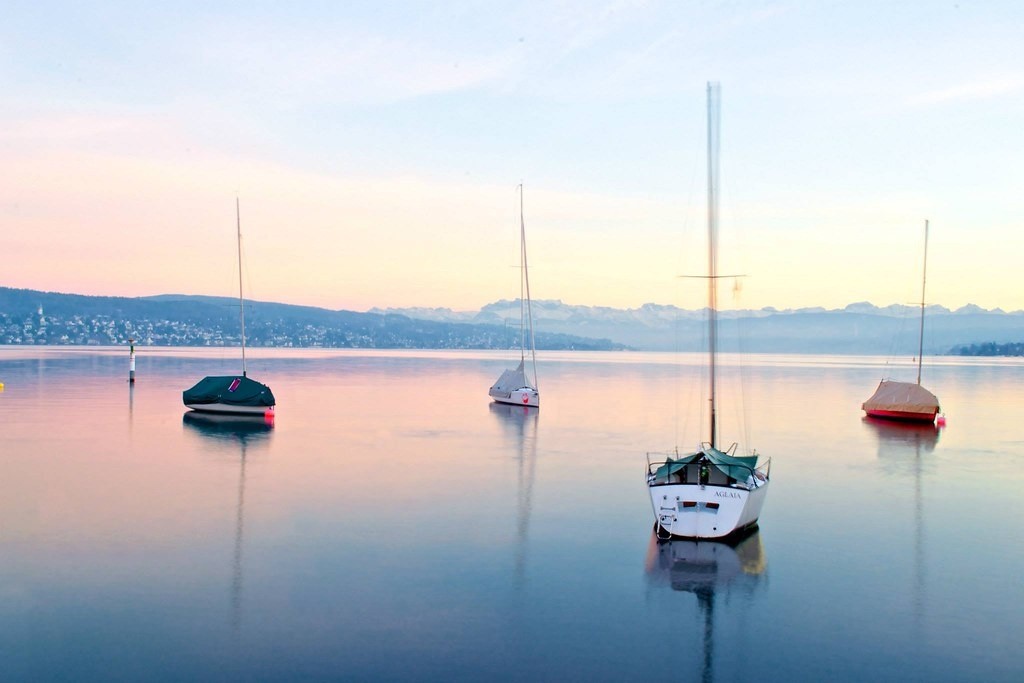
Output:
[643,81,773,540]
[488,182,543,408]
[181,195,276,417]
[861,219,948,430]
[646,525,767,682]
